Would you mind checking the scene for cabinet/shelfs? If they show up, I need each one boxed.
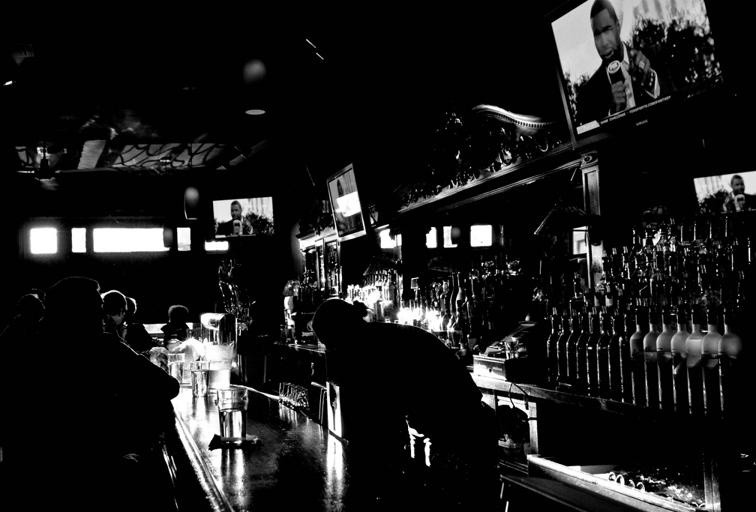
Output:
[295,230,363,350]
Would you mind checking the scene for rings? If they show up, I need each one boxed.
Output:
[638,60,647,70]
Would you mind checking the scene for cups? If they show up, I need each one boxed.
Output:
[164,350,246,449]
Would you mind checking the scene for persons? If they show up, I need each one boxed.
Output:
[215,200,256,236]
[306,299,505,511]
[571,0,686,127]
[724,175,756,215]
[0,270,191,512]
[334,179,362,237]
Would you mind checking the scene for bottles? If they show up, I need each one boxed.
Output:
[546,214,756,409]
[401,267,482,352]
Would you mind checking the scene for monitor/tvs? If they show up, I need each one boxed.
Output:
[691,169,756,217]
[325,162,369,243]
[537,0,727,152]
[211,195,277,241]
